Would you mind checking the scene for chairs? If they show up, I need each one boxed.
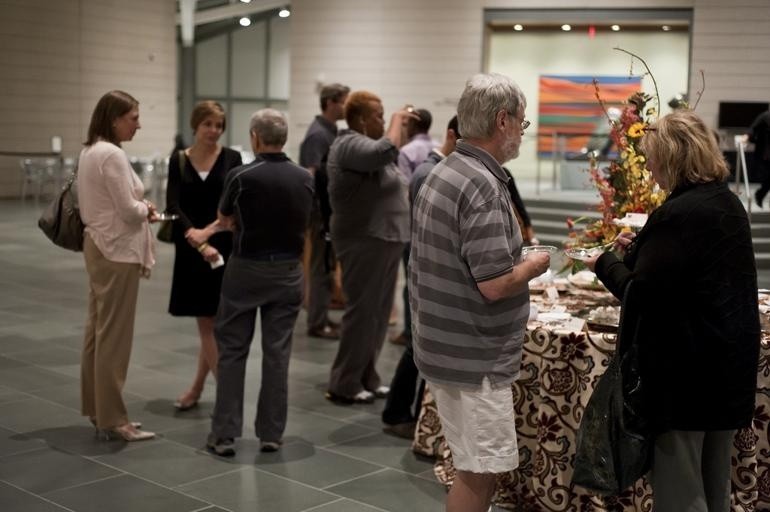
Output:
[16,154,175,229]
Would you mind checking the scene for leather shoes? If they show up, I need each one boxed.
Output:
[329,321,340,329]
[383,424,415,438]
[174,398,197,410]
[309,328,340,340]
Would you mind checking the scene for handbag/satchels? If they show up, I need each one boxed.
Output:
[156,150,185,244]
[37,153,84,253]
[571,282,651,496]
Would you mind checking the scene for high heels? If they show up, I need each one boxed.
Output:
[89,415,141,432]
[105,426,154,441]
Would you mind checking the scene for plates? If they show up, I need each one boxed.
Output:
[521,244,557,260]
[566,246,594,261]
[568,275,602,291]
[527,284,569,295]
[152,213,179,222]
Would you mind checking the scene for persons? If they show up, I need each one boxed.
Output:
[747,109,770,207]
[76,90,159,441]
[408,74,551,512]
[582,110,762,512]
[500,164,535,246]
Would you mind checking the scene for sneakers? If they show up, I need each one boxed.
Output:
[371,385,390,397]
[260,438,283,452]
[325,389,376,404]
[208,433,236,456]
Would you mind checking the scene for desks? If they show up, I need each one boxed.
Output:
[411,284,768,512]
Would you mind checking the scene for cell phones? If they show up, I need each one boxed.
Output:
[155,214,179,221]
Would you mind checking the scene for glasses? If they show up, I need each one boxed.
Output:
[509,112,530,129]
